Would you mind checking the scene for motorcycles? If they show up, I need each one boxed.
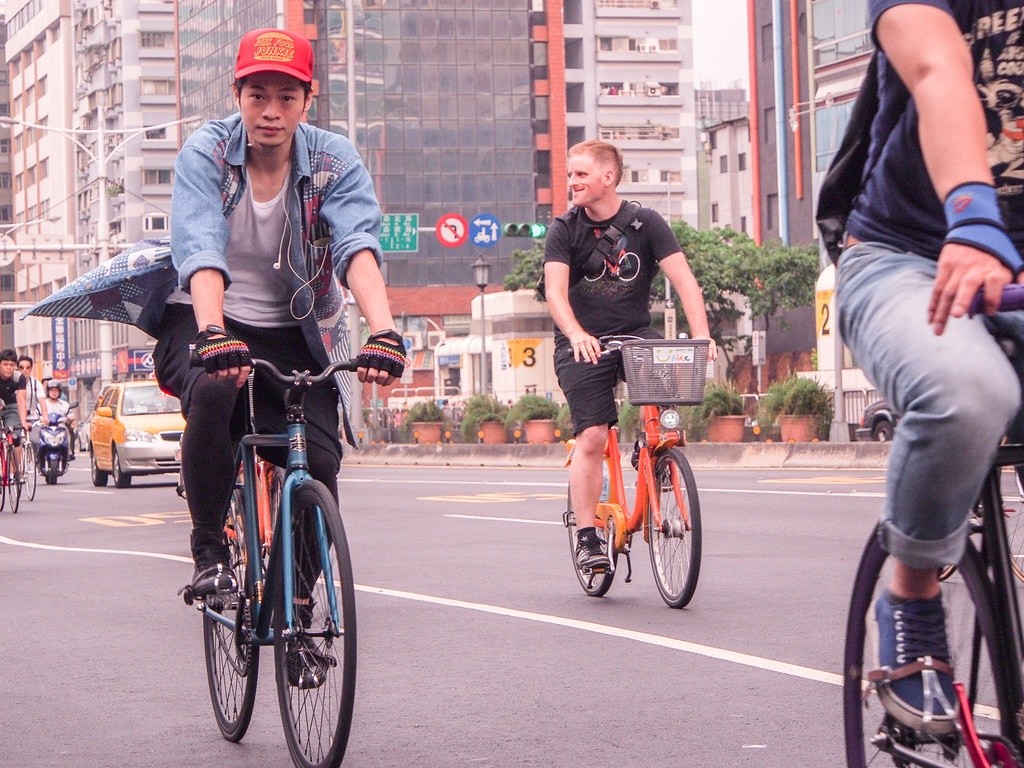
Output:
[33,401,79,485]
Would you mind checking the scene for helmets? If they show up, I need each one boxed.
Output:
[46,379,63,397]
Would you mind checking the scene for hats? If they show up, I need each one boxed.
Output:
[235,27,314,84]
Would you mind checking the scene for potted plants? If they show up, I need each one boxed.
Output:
[460,394,513,444]
[759,375,836,443]
[400,400,451,444]
[505,394,558,444]
[694,379,748,441]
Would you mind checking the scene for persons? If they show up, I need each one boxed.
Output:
[836,1,1023,734]
[543,141,719,568]
[0,347,76,495]
[151,28,406,686]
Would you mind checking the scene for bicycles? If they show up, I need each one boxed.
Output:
[559,332,750,607]
[184,355,377,767]
[0,415,39,513]
[839,277,1024,768]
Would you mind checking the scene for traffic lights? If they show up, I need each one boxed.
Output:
[502,222,548,239]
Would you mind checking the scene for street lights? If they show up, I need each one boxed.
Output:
[1,112,203,408]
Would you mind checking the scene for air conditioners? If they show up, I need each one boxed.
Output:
[648,88,662,96]
[402,330,447,350]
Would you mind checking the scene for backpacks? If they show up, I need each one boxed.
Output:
[815,0,986,269]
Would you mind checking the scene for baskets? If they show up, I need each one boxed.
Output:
[619,339,711,406]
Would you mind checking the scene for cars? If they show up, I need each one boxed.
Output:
[852,392,903,443]
[88,380,193,488]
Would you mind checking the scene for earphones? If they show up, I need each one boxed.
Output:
[273,255,281,270]
[237,98,239,105]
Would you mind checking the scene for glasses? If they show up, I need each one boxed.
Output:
[19,365,31,369]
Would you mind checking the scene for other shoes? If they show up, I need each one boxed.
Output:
[13,472,25,483]
[67,455,71,460]
[71,453,75,460]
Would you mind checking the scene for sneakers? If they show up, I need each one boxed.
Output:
[632,440,675,493]
[867,586,959,735]
[285,595,328,689]
[189,525,232,596]
[575,531,612,569]
